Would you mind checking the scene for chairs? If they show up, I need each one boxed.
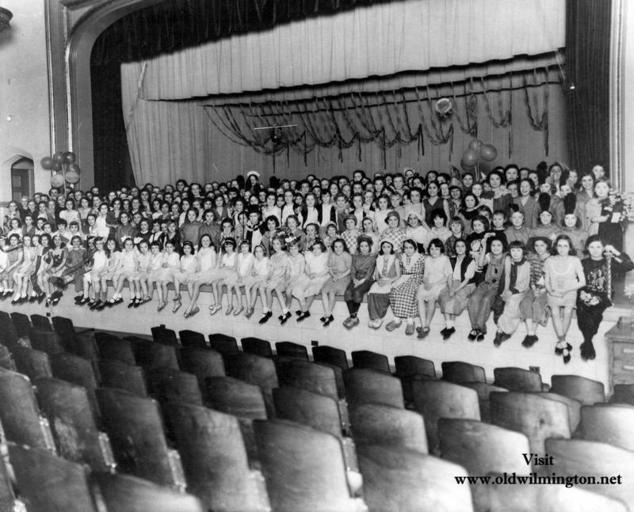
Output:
[0,310,634,512]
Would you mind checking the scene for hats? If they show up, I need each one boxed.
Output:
[342,214,358,225]
[383,211,400,224]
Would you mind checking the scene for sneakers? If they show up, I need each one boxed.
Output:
[404,321,416,335]
[416,326,432,338]
[367,316,384,330]
[491,331,516,346]
[319,313,335,327]
[342,314,360,329]
[277,310,291,323]
[0,287,274,326]
[520,334,540,347]
[554,341,574,364]
[465,329,487,341]
[385,321,403,332]
[294,310,311,322]
[438,327,457,340]
[580,343,597,359]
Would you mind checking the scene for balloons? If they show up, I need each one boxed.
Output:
[63,151,76,164]
[52,153,64,165]
[460,158,474,173]
[40,158,53,169]
[463,150,477,167]
[480,144,497,161]
[468,139,484,161]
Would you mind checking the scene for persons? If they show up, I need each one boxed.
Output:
[0,160,634,364]
